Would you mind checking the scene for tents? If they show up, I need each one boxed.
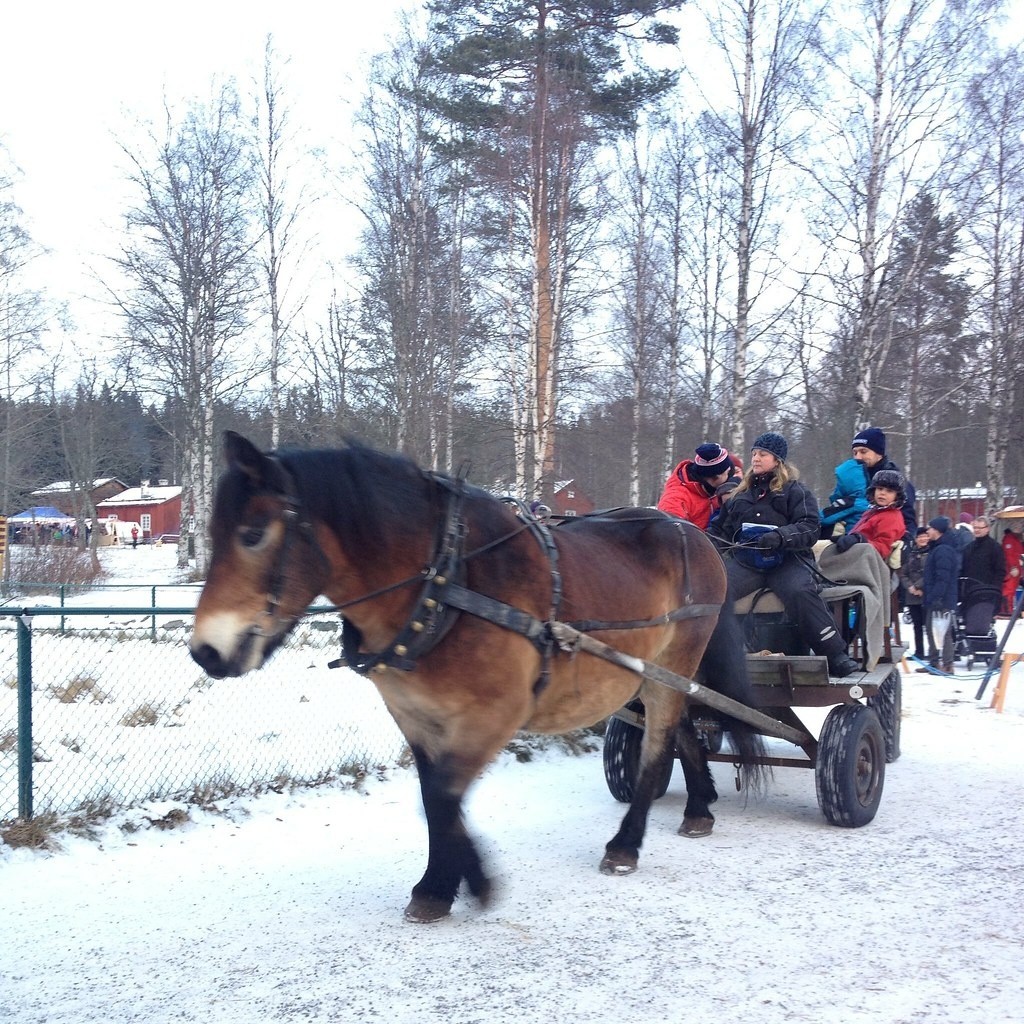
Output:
[7,506,75,535]
[64,518,144,546]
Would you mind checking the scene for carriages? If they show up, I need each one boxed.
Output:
[187,429,911,923]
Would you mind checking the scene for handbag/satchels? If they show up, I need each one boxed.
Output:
[733,527,786,570]
[932,610,951,650]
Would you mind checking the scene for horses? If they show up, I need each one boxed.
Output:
[188,429,777,924]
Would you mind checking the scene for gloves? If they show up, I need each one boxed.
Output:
[830,522,847,543]
[888,532,917,569]
[759,531,781,549]
[837,533,867,553]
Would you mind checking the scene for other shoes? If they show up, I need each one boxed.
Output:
[828,651,858,678]
[906,654,924,661]
[929,664,954,676]
[915,664,940,673]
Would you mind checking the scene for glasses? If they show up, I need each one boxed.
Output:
[973,525,987,529]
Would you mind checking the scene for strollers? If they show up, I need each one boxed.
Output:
[952,577,1003,672]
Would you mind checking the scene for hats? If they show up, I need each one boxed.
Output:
[851,428,886,455]
[960,512,973,524]
[692,442,730,476]
[867,470,906,508]
[728,453,743,468]
[928,517,948,533]
[716,482,738,505]
[750,431,787,464]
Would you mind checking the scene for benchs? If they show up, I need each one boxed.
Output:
[732,539,903,670]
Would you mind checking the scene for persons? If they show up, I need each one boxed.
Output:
[702,431,860,678]
[952,515,1007,662]
[819,428,918,578]
[1000,520,1024,613]
[898,527,933,661]
[819,459,872,537]
[656,441,745,537]
[836,469,908,561]
[131,524,139,549]
[952,511,977,577]
[915,516,960,676]
[7,522,108,549]
